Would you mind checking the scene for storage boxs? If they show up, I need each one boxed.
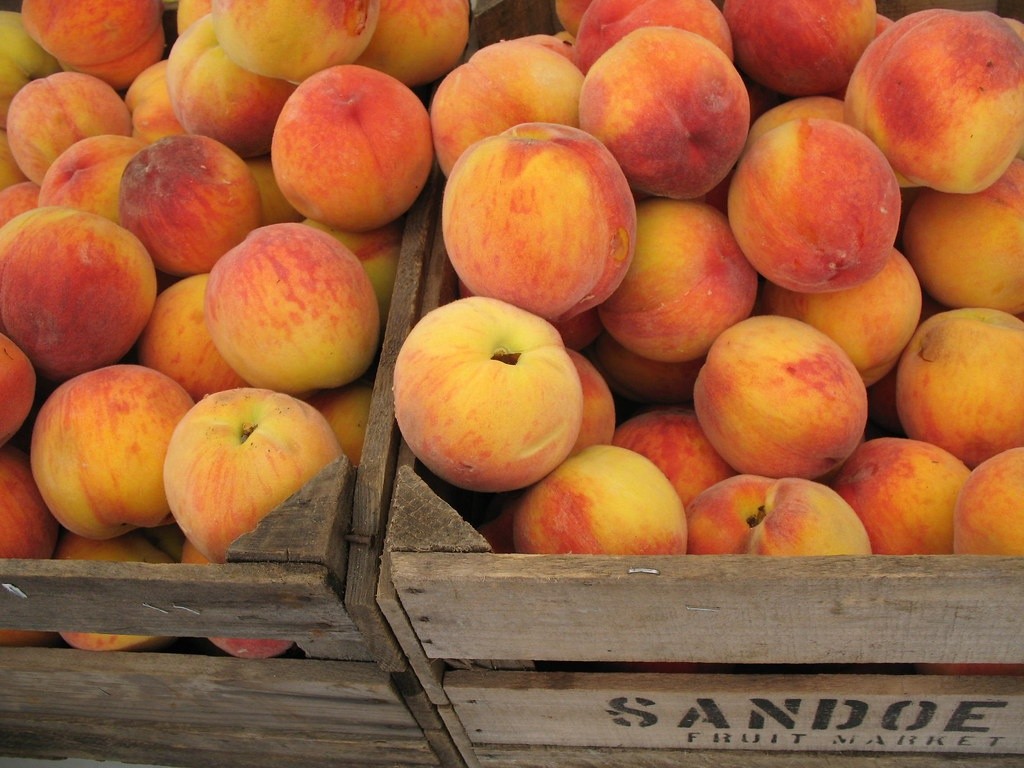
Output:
[0,0,1024,768]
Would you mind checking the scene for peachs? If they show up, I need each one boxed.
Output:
[0,0,1024,677]
